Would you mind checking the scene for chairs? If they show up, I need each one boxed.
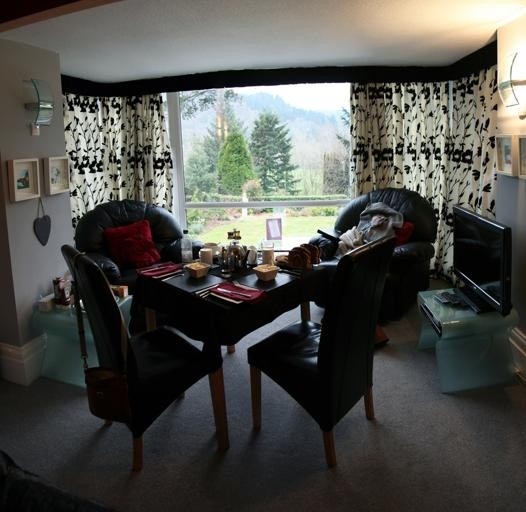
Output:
[75,199,203,336]
[314,188,437,325]
[61,242,222,471]
[248,233,397,469]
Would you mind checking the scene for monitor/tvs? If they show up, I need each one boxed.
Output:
[452,204,513,317]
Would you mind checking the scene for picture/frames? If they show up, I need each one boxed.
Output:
[7,158,41,202]
[43,156,69,196]
[495,134,517,177]
[518,135,526,179]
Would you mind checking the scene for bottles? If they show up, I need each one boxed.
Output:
[181,230,192,263]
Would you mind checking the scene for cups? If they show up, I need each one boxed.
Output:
[200,249,213,266]
[261,243,275,265]
[247,246,258,265]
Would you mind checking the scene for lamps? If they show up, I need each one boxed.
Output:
[24,78,54,127]
[497,52,526,108]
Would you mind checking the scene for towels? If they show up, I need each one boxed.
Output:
[134,260,181,277]
[209,282,267,303]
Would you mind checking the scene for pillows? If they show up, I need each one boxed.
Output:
[104,219,160,269]
[393,222,414,247]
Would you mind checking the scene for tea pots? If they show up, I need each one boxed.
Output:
[198,243,222,259]
[222,242,250,262]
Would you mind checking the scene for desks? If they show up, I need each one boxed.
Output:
[417,288,520,393]
[32,293,133,389]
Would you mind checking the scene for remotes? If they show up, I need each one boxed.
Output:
[455,294,468,308]
[441,292,460,305]
[433,293,451,304]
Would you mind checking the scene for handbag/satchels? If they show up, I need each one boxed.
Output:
[85,367,126,421]
